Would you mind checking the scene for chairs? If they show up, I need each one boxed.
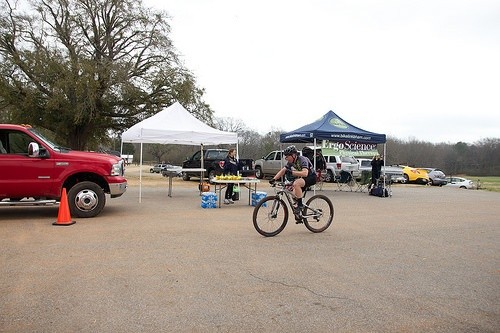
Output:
[334,171,370,193]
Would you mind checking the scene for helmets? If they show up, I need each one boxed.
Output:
[283,145,297,156]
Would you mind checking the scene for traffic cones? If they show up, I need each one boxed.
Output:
[52,188,76,226]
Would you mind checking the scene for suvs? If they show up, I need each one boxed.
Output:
[254,150,302,179]
[182,148,230,184]
[0,123,127,218]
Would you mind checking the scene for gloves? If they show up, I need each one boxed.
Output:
[285,169,291,175]
[269,178,276,184]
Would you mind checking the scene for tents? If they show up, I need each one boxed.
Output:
[279,110,386,198]
[120,100,239,203]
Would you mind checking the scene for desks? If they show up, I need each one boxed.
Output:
[163,168,207,198]
[211,179,260,208]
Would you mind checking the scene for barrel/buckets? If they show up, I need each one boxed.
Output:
[232,186,241,201]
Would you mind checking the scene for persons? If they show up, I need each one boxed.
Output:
[222,149,246,204]
[269,145,317,222]
[368,155,384,191]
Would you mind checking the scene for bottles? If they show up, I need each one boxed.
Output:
[293,203,298,209]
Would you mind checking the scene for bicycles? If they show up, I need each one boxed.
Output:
[252,179,334,237]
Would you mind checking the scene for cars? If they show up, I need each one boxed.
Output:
[446,177,474,189]
[381,166,403,184]
[324,155,359,182]
[357,158,373,179]
[423,168,447,186]
[163,166,181,177]
[150,164,166,173]
[401,166,429,185]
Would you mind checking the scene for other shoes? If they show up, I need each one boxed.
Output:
[223,199,229,204]
[228,198,234,204]
[293,206,303,214]
